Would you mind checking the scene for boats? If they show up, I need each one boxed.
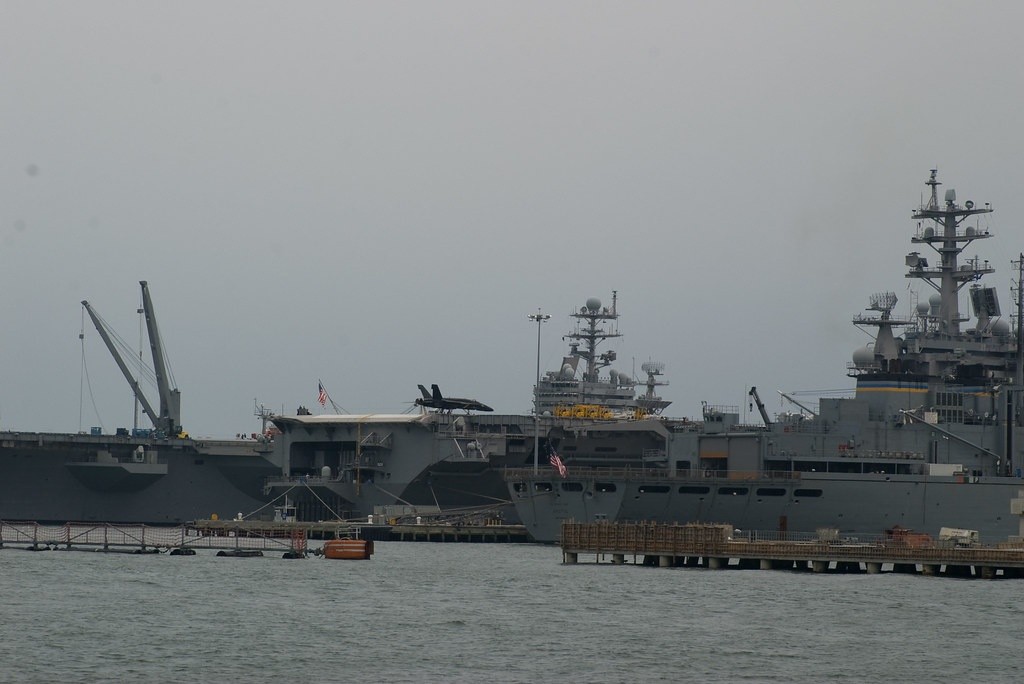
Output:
[252,290,764,535]
[489,165,1024,551]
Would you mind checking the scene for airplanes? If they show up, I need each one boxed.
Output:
[413,383,494,414]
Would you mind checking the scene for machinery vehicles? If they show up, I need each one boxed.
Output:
[80,279,189,440]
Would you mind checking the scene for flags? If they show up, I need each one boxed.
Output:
[318,384,327,405]
[548,444,567,477]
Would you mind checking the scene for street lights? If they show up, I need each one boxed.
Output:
[527,306,550,475]
